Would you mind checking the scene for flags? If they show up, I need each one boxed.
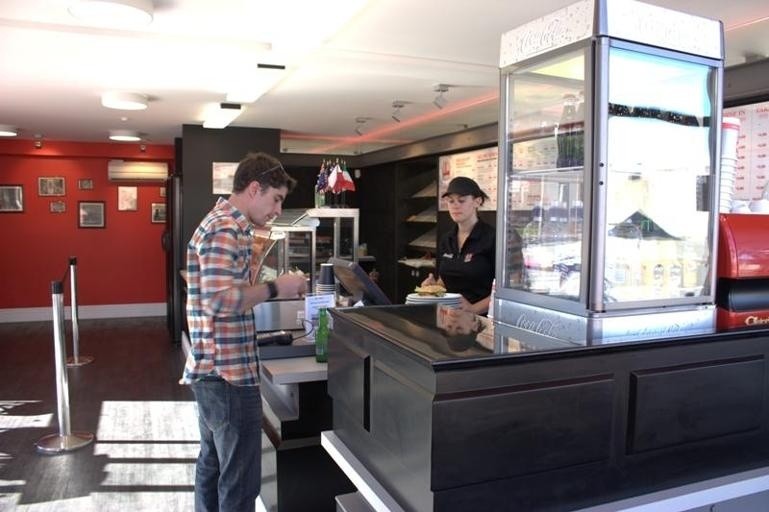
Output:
[315,162,356,195]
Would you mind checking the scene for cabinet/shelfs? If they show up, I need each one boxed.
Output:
[321,301,769,512]
[394,156,438,301]
[493,0,725,345]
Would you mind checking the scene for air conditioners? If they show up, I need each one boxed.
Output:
[107,159,168,183]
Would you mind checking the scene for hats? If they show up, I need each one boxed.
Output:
[441,177,487,199]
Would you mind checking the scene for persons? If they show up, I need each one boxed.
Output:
[523,206,546,237]
[440,307,486,353]
[420,177,524,314]
[178,152,310,511]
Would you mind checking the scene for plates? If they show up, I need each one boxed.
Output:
[404,292,463,309]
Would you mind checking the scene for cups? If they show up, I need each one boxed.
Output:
[719,117,741,215]
[315,263,335,292]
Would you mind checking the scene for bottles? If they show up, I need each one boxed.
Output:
[573,91,584,166]
[515,203,584,296]
[556,93,576,168]
[315,306,330,362]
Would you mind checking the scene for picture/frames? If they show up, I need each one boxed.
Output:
[0,177,167,229]
[209,160,240,196]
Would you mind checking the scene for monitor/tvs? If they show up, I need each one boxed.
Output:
[326,257,392,306]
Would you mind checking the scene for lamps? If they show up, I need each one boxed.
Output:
[107,130,143,141]
[356,125,365,135]
[202,101,244,129]
[226,64,285,104]
[392,109,402,123]
[0,124,17,137]
[432,96,447,109]
[101,92,148,111]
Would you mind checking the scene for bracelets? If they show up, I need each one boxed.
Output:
[265,280,277,299]
